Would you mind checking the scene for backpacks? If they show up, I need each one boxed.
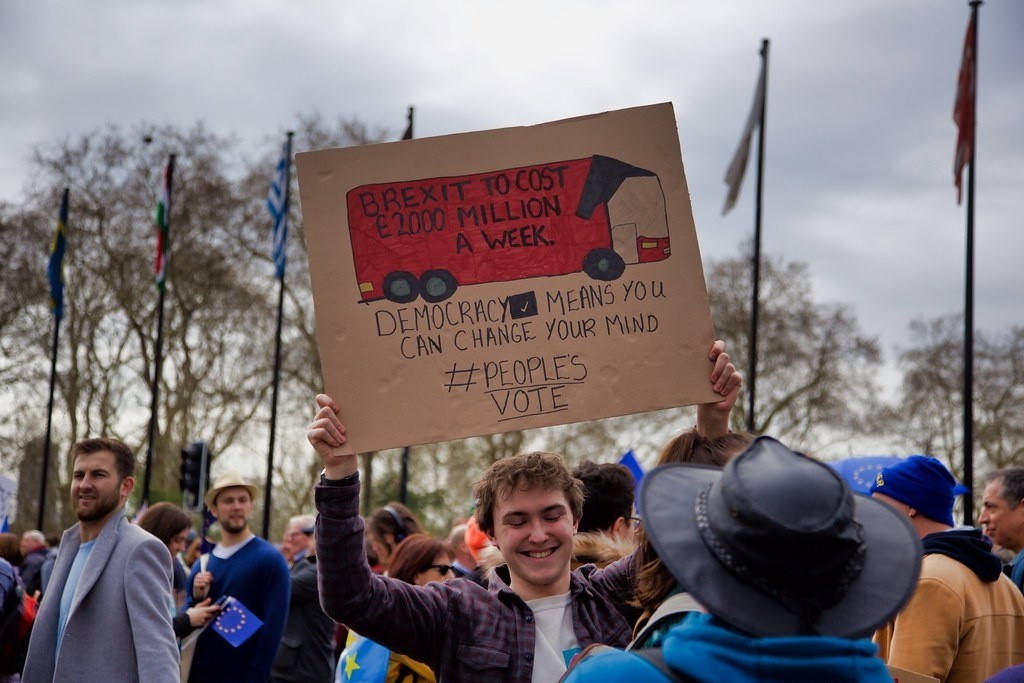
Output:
[0,568,36,642]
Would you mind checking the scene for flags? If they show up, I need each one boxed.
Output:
[45,189,68,322]
[720,65,765,218]
[152,156,173,291]
[265,140,291,279]
[951,15,978,204]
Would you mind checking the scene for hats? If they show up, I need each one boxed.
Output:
[639,435,923,639]
[869,454,970,528]
[204,475,258,509]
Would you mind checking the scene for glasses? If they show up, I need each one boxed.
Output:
[418,564,455,575]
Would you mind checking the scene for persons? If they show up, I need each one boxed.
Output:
[0,339,1024,683]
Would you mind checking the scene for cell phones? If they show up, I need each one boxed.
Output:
[215,595,226,606]
